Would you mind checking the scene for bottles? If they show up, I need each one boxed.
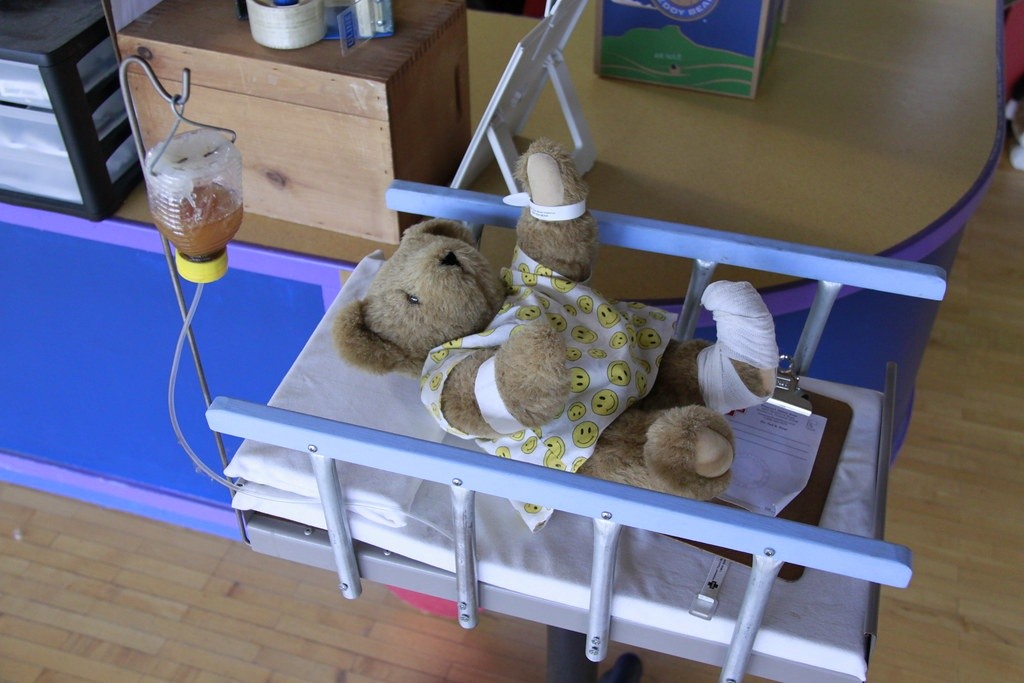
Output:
[145,129,244,284]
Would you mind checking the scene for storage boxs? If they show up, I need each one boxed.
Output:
[594,0,784,101]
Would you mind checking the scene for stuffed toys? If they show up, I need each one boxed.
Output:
[332,136,779,533]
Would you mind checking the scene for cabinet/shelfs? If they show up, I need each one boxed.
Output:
[115,1,471,245]
[0,0,143,223]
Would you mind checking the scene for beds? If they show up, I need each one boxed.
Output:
[206,181,948,683]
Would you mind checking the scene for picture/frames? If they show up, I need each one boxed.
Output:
[449,0,595,196]
